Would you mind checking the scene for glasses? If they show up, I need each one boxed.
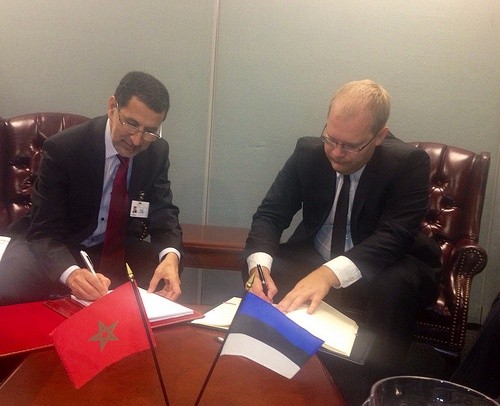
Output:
[321,124,376,153]
[117,109,163,141]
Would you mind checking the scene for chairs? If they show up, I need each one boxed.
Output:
[385,142,489,377]
[1,113,96,238]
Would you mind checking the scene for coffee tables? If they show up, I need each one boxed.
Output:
[180,223,250,272]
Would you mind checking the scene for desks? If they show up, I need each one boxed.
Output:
[0,304,344,406]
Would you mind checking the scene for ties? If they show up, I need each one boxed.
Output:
[331,175,350,258]
[98,154,130,288]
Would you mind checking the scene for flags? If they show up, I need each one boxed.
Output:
[49,281,156,388]
[219,291,325,380]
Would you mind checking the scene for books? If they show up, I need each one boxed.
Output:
[187,296,379,365]
[0,287,205,358]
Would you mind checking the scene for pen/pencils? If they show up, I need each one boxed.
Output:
[80,249,100,275]
[257,264,271,296]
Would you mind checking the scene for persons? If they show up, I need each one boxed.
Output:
[244,80,443,388]
[0,72,181,300]
[471,292,500,395]
